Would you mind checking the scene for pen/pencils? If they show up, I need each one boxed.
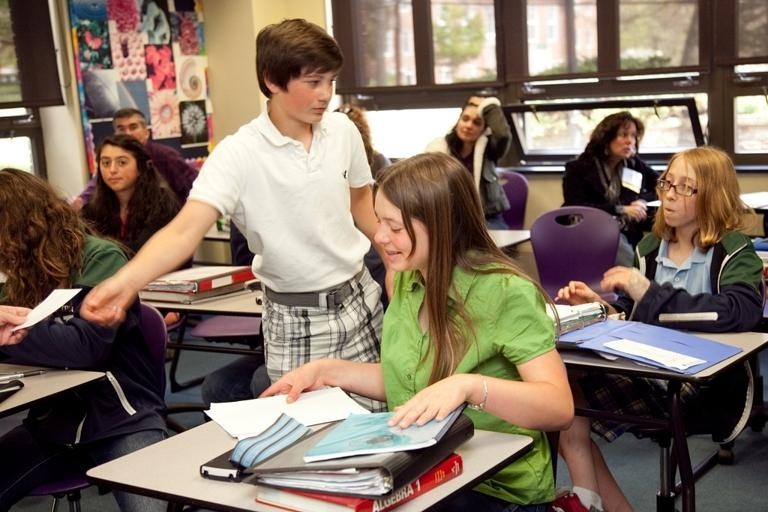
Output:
[0,370,48,381]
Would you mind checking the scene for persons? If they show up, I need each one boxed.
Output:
[80,136,196,273]
[421,89,512,229]
[255,151,580,512]
[69,109,201,210]
[81,17,399,415]
[553,146,767,510]
[333,106,390,176]
[561,110,660,244]
[0,303,39,348]
[0,167,173,511]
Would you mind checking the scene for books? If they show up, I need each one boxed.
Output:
[255,454,465,512]
[301,401,468,465]
[137,282,246,304]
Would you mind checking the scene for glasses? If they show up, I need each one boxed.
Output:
[656,178,698,197]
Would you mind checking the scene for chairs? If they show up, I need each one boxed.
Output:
[23,300,169,511]
[228,230,252,267]
[492,170,527,254]
[169,308,262,395]
[528,204,620,305]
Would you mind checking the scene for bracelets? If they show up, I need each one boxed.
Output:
[465,372,488,412]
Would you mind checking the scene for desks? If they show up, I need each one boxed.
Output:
[485,227,531,251]
[549,315,767,512]
[86,412,534,512]
[643,191,766,215]
[0,362,107,419]
[191,222,233,265]
[138,287,263,393]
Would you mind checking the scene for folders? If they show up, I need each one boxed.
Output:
[0,378,25,404]
[243,409,475,501]
[554,302,744,376]
[143,266,256,294]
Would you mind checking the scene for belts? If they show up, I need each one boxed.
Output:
[267,267,366,308]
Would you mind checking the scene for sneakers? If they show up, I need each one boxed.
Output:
[553,491,595,511]
